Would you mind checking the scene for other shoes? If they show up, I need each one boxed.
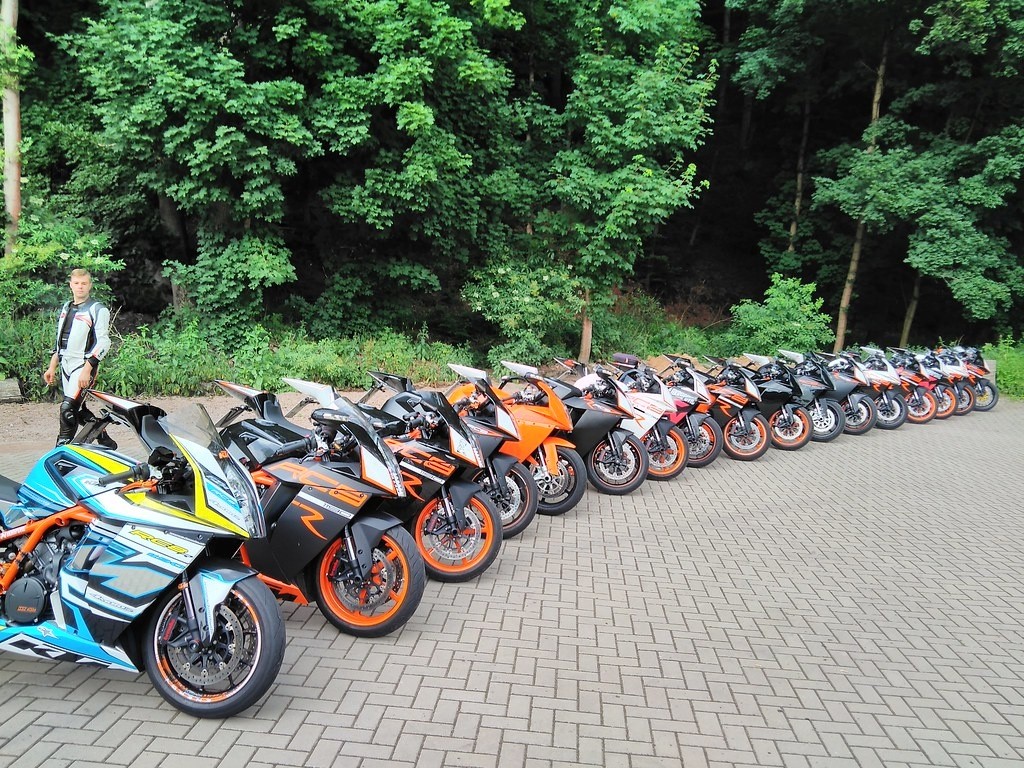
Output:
[97,435,118,451]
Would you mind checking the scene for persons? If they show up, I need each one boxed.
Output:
[44,268,117,450]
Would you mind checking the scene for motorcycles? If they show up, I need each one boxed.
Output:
[0,387,287,719]
[211,379,504,573]
[84,380,428,639]
[286,345,999,538]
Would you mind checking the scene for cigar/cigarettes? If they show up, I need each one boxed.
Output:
[46,382,50,387]
